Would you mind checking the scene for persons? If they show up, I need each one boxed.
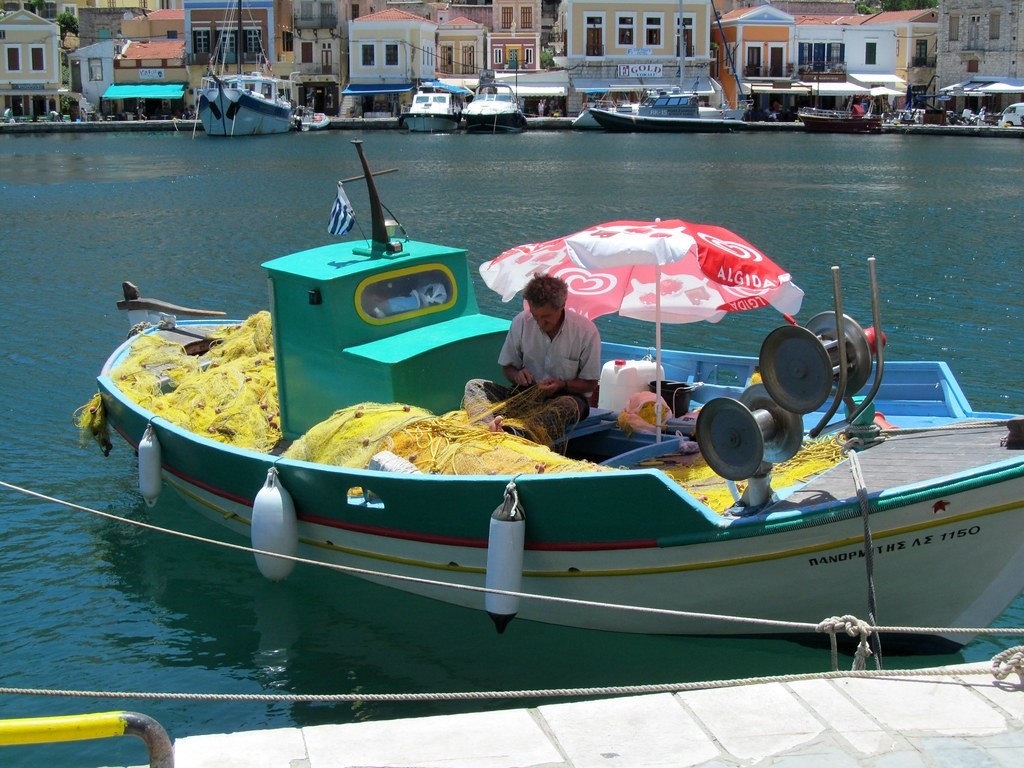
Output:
[137,103,190,120]
[537,98,558,118]
[946,106,986,125]
[753,101,784,122]
[4,107,88,122]
[881,110,923,124]
[466,274,602,444]
[279,94,314,122]
[722,101,729,120]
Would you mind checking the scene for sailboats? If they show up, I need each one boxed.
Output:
[571,0,753,129]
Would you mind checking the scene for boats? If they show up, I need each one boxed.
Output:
[459,81,529,134]
[98,136,1024,655]
[290,106,331,131]
[195,0,292,136]
[796,107,882,134]
[587,89,805,132]
[401,89,461,133]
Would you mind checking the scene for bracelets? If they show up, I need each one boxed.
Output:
[564,381,568,388]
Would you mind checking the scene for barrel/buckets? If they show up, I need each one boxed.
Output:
[597,358,665,418]
[647,380,693,418]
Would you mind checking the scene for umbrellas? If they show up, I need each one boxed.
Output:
[481,218,803,442]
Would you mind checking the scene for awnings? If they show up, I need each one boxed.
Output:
[102,85,186,101]
[438,77,715,95]
[343,84,416,95]
[939,78,1024,97]
[743,74,908,97]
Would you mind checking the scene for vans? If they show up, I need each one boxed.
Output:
[999,104,1024,127]
